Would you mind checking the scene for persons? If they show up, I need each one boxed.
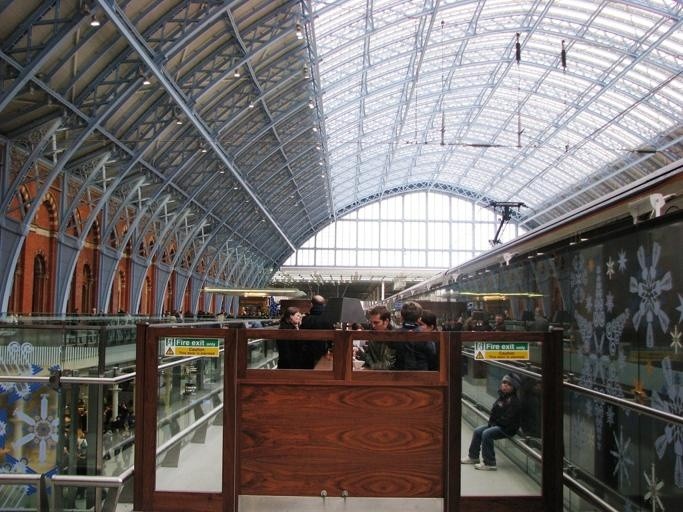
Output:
[69,308,79,344]
[64,399,135,498]
[278,295,331,369]
[441,315,505,331]
[351,302,440,370]
[117,308,127,317]
[90,308,98,316]
[165,310,227,323]
[461,373,524,471]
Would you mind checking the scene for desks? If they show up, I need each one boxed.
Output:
[312,348,370,370]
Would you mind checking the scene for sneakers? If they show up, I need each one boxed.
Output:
[461,455,480,464]
[475,462,497,471]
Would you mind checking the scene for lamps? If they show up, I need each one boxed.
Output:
[320,297,368,330]
[243,194,249,202]
[173,116,183,125]
[233,183,239,189]
[91,12,101,28]
[140,72,151,86]
[198,141,207,152]
[248,98,254,107]
[218,165,225,173]
[235,68,241,77]
[289,7,333,222]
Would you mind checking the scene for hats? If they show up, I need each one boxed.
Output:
[502,372,521,390]
